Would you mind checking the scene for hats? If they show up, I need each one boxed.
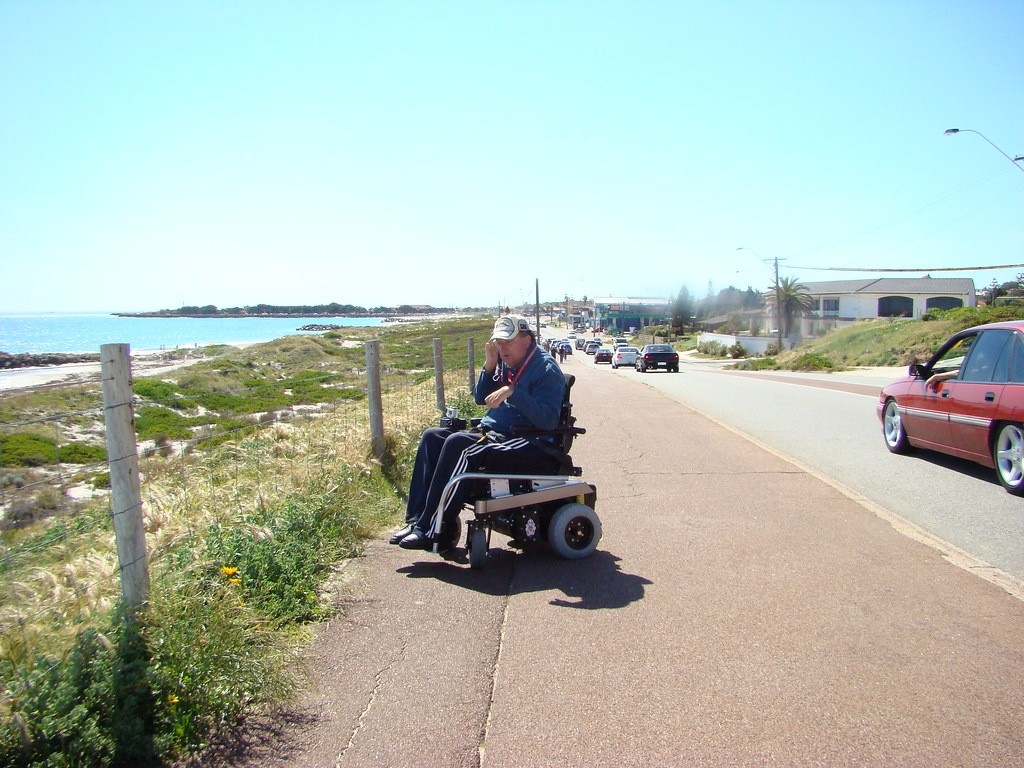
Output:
[488,314,530,344]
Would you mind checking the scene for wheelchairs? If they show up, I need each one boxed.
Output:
[440,374,602,568]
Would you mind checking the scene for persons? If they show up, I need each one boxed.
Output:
[388,314,566,550]
[542,338,566,365]
[924,348,992,393]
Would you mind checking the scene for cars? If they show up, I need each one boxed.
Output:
[876,321,1024,497]
[635,344,679,373]
[531,320,546,328]
[548,328,604,355]
[594,349,613,364]
[611,347,641,370]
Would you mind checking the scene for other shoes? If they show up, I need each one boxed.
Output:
[399,532,432,549]
[390,521,416,545]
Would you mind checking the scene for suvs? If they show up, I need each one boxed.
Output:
[613,338,628,351]
